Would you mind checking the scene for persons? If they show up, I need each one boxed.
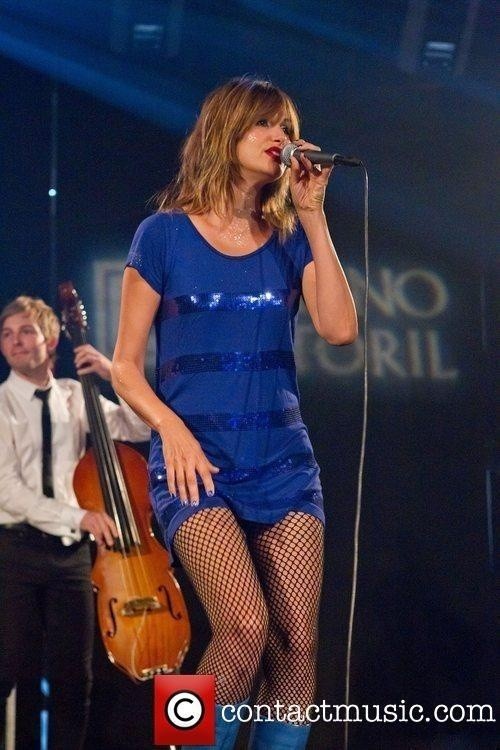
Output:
[111,74,359,750]
[0,295,154,750]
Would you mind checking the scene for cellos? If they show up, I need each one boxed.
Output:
[58,280,190,681]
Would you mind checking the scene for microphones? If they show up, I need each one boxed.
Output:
[279,143,363,167]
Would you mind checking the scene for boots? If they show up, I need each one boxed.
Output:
[181,695,312,750]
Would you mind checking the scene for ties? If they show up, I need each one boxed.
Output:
[34,386,55,498]
